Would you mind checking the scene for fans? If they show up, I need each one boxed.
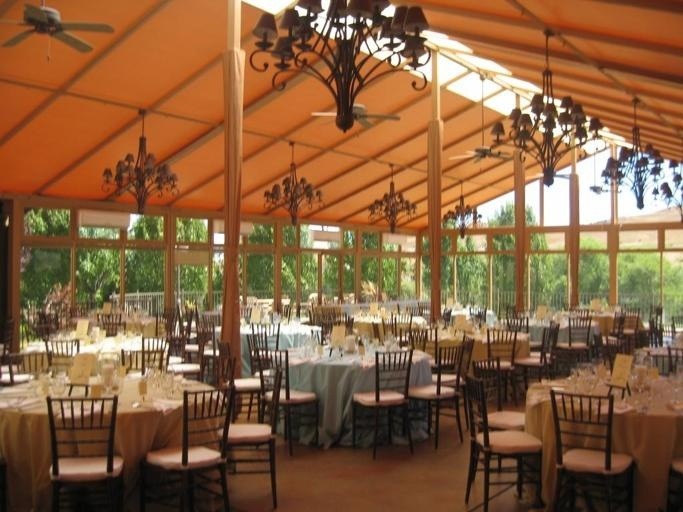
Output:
[311,104,399,129]
[0,0,112,65]
[447,76,515,163]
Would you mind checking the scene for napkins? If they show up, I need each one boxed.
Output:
[7,398,43,410]
[57,401,99,422]
[151,396,180,414]
[598,402,633,414]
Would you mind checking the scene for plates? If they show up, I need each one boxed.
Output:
[602,402,634,415]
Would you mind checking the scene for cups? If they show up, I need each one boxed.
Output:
[109,377,121,395]
[52,374,66,396]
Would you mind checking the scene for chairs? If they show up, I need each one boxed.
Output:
[254,346,318,454]
[219,364,281,509]
[141,386,233,512]
[46,394,125,510]
[463,375,542,509]
[350,351,412,460]
[472,357,528,475]
[664,459,681,511]
[3,288,682,392]
[407,343,463,451]
[549,388,634,512]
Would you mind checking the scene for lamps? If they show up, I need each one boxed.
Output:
[605,97,682,209]
[263,141,327,227]
[249,1,432,133]
[366,165,416,234]
[33,21,62,62]
[442,180,482,239]
[478,30,605,185]
[100,110,180,215]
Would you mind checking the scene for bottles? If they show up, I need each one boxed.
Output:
[358,335,365,356]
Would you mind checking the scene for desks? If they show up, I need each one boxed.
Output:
[276,349,431,445]
[0,375,227,510]
[525,375,682,508]
